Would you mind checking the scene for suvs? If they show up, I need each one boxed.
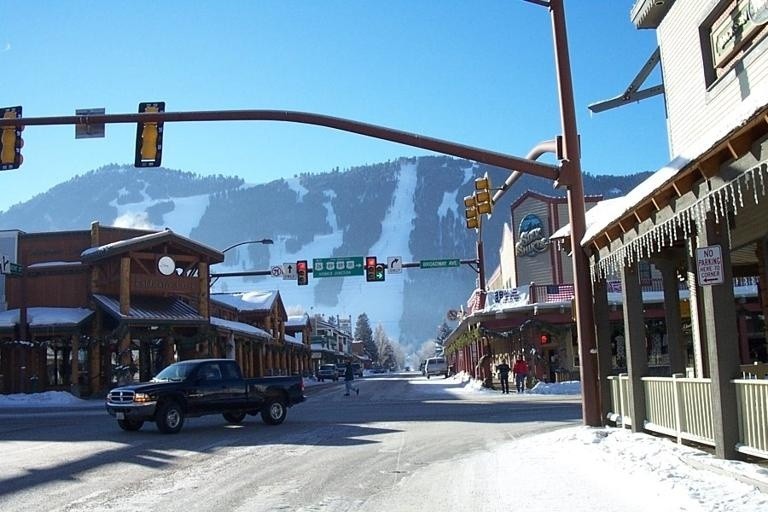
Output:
[317,363,338,383]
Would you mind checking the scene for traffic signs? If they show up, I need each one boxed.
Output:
[282,261,297,281]
[311,255,364,278]
[10,262,24,274]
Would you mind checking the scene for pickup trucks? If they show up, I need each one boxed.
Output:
[104,358,308,434]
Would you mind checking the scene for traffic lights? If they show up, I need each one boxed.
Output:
[364,255,376,282]
[375,262,385,282]
[386,255,402,275]
[462,190,481,229]
[473,170,493,220]
[419,257,460,269]
[296,259,308,286]
[540,334,548,344]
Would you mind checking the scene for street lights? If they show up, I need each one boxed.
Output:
[220,238,274,252]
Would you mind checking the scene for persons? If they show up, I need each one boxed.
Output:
[495,359,512,395]
[342,363,360,396]
[512,354,529,394]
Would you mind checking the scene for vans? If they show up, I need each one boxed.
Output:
[424,357,447,379]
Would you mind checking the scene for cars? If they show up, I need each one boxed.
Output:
[350,364,362,377]
[405,367,409,371]
[419,362,426,375]
[337,364,347,377]
[390,366,394,371]
[374,367,384,373]
